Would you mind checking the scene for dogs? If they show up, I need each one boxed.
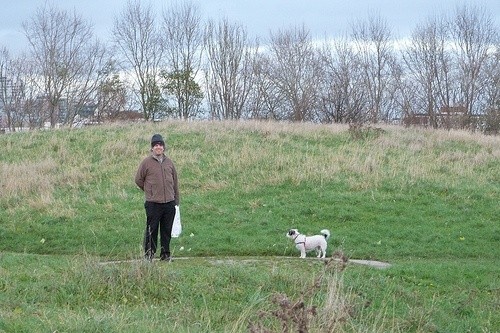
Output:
[286,228,330,258]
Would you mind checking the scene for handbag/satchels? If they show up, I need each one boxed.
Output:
[171,205,182,237]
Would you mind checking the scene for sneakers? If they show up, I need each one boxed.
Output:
[160,256,175,262]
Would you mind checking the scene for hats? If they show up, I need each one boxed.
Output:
[151,134,165,146]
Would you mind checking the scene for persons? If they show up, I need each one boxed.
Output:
[134,134,179,263]
